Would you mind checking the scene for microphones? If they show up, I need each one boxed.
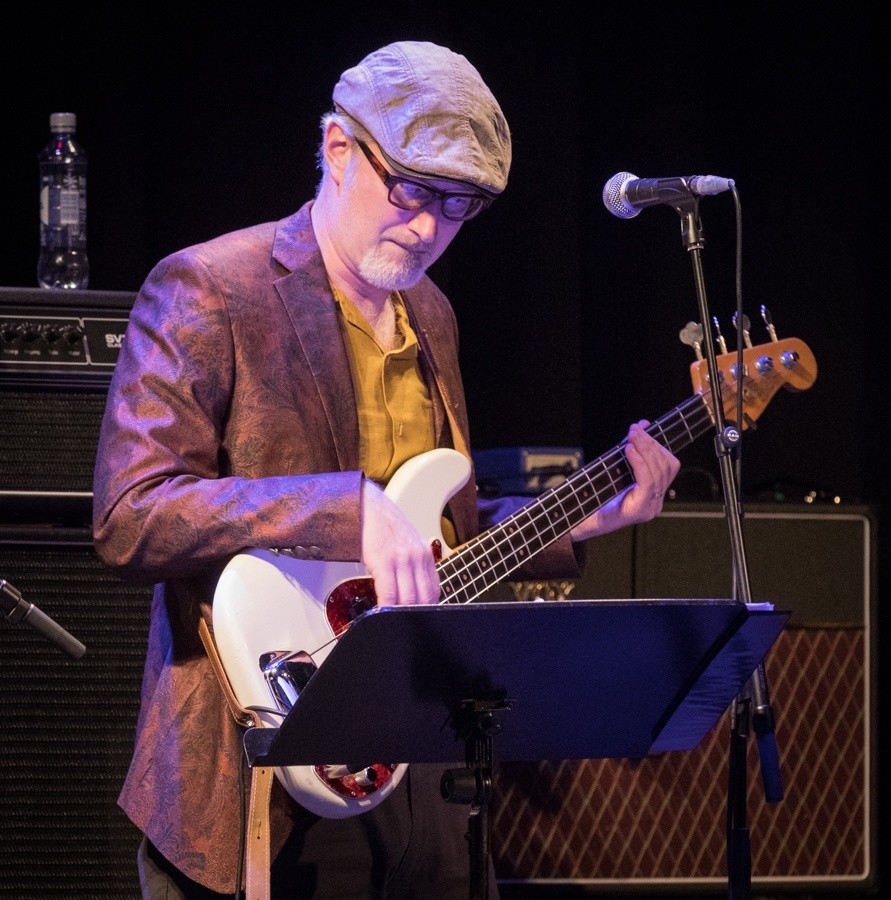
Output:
[602,171,736,218]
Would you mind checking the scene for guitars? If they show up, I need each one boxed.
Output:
[210,304,818,820]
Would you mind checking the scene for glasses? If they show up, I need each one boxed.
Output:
[354,134,495,222]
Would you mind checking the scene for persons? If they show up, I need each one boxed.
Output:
[92,41,680,900]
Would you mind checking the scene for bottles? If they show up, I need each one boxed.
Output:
[41,113,89,289]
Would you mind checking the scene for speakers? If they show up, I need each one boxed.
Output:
[0,516,156,900]
[468,501,880,890]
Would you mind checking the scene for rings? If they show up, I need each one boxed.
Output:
[652,492,663,499]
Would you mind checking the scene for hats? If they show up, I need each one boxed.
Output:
[332,41,512,193]
